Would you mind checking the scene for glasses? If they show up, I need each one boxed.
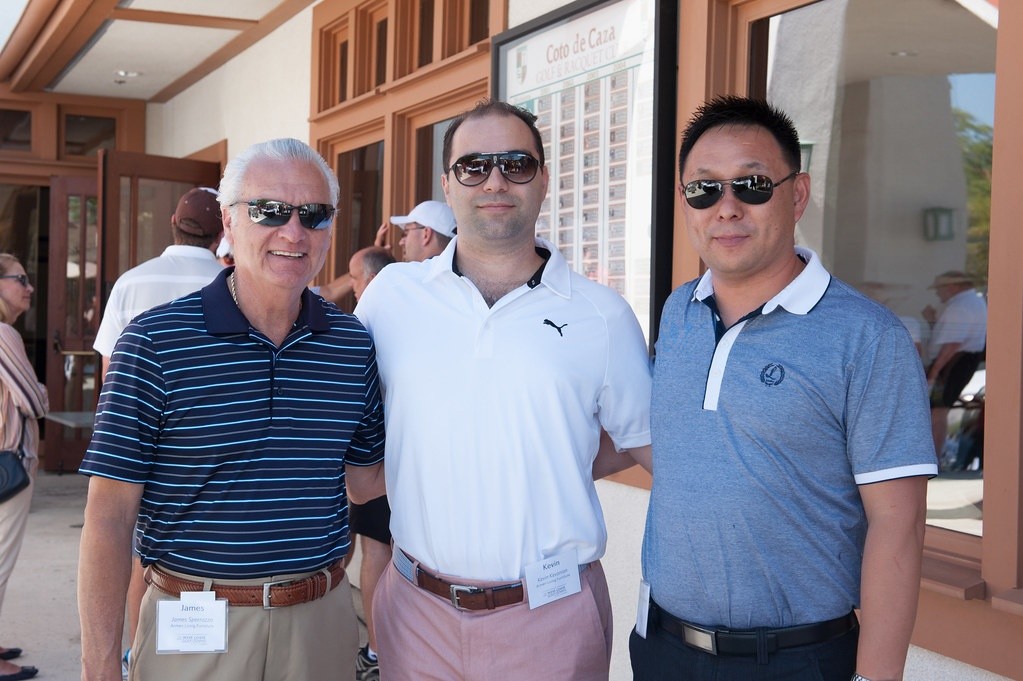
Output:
[401,226,426,240]
[0,272,33,288]
[681,169,800,210]
[227,197,334,230]
[447,150,545,186]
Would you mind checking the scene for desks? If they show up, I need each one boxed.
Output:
[45,411,95,474]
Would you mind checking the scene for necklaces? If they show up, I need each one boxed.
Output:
[231,272,240,309]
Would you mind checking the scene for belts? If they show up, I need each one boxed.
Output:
[391,542,589,612]
[145,560,345,610]
[650,604,860,655]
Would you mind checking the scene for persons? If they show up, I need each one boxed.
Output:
[217,222,391,302]
[593,95,938,681]
[77,138,385,681]
[925,272,987,458]
[352,101,654,681]
[0,252,49,681]
[93,188,225,681]
[348,201,457,681]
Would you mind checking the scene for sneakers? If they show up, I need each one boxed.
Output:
[354,642,381,681]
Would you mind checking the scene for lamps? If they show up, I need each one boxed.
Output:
[923,206,957,241]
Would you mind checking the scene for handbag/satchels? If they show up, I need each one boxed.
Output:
[0,450,30,505]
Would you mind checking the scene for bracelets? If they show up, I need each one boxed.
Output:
[851,672,870,681]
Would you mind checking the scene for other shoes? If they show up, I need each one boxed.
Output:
[0,664,39,681]
[125,647,134,666]
[0,647,21,660]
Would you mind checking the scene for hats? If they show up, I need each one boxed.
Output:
[175,186,224,236]
[388,200,458,240]
[215,234,234,259]
